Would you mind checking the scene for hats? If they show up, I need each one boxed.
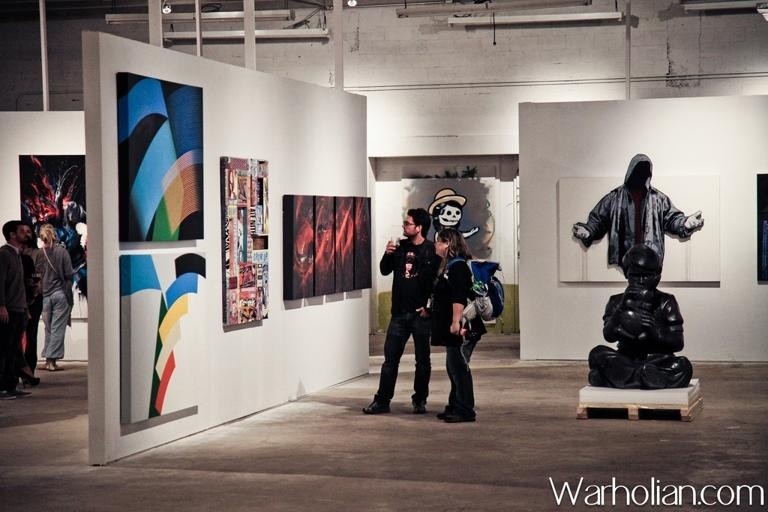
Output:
[428,186,467,219]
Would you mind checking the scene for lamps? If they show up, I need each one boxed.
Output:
[448,12,624,28]
[103,9,291,24]
[163,9,332,42]
[681,2,767,14]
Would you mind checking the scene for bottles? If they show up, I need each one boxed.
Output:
[471,279,483,291]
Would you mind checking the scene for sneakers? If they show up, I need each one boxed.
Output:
[411,400,426,414]
[360,395,392,415]
[436,409,476,423]
[2,360,69,402]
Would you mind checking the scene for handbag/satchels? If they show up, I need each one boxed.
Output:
[444,256,506,325]
[64,282,78,306]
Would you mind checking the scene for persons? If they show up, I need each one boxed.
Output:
[571,154,703,266]
[0,221,76,400]
[364,207,442,414]
[430,228,487,423]
[588,244,693,388]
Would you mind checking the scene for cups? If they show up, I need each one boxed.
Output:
[390,236,400,249]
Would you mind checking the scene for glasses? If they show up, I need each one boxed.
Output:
[400,220,417,228]
